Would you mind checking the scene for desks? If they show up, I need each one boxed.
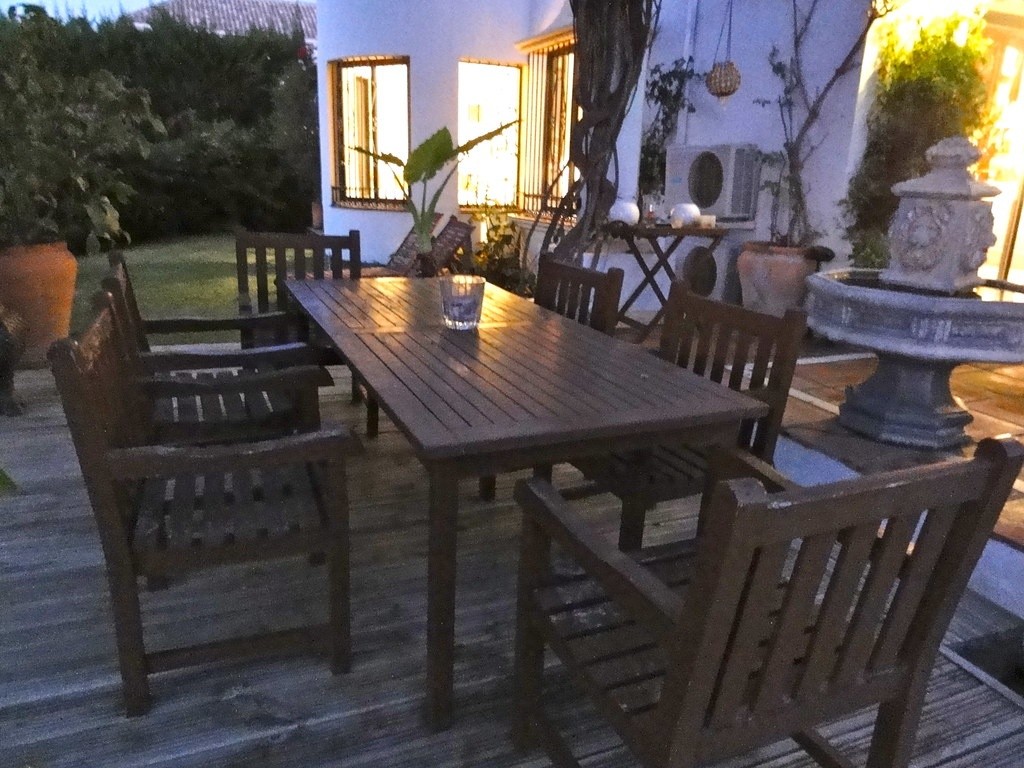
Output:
[283,274,772,736]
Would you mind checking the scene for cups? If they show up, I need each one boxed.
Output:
[440,274,485,331]
[698,215,716,228]
[643,194,656,229]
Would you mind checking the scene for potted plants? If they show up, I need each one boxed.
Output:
[0,4,187,373]
[735,40,830,319]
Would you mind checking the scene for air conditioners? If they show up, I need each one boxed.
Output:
[675,235,745,309]
[664,143,764,223]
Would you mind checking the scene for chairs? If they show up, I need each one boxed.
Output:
[361,215,478,278]
[49,289,366,718]
[101,251,318,450]
[533,280,812,554]
[480,253,624,505]
[287,212,445,279]
[510,435,1024,768]
[237,228,362,411]
